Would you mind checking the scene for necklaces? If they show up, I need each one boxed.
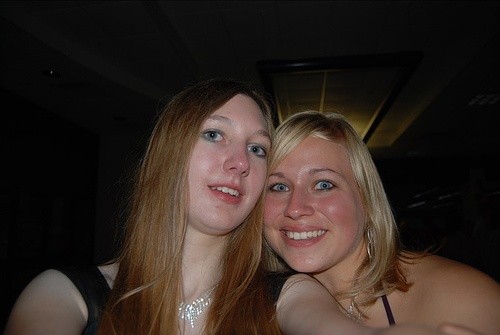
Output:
[178,284,215,329]
[343,296,371,322]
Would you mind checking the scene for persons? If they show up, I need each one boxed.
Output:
[261,111,500,335]
[3,75,479,335]
